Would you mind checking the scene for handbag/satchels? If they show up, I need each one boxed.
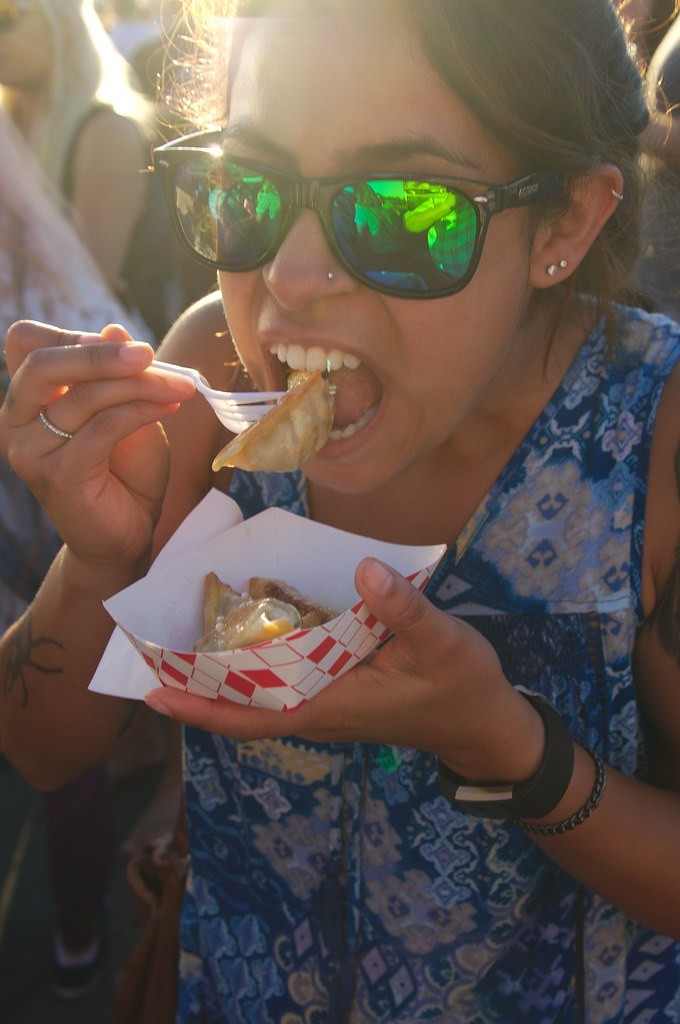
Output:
[111,832,191,1024]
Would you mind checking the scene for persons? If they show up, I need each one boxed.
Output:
[0,0,680,1024]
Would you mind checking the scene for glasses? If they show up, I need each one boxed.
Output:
[153,128,568,299]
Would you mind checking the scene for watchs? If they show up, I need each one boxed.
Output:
[438,685,574,821]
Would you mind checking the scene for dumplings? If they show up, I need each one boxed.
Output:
[191,571,346,656]
[212,368,336,473]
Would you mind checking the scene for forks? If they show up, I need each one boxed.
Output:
[149,359,285,434]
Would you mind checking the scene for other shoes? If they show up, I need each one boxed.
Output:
[50,903,110,998]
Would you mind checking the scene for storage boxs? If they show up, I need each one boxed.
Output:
[99,507,448,710]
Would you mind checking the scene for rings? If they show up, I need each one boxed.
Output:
[38,407,71,438]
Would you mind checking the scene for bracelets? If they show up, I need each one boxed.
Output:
[505,741,606,836]
